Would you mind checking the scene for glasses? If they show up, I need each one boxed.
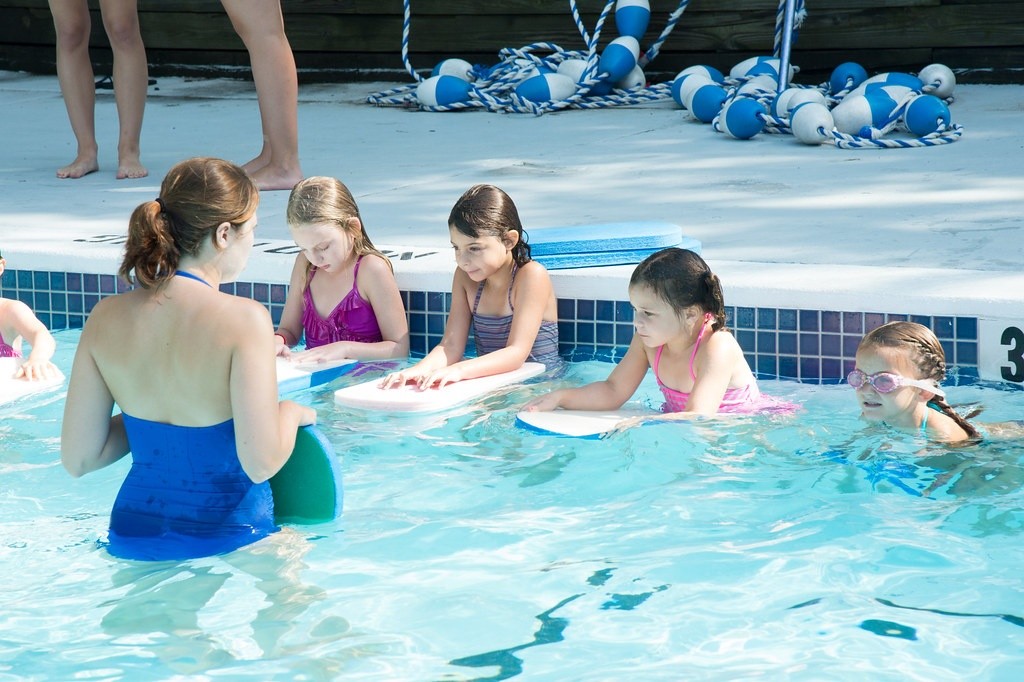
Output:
[846,370,946,400]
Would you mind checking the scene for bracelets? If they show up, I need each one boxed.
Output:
[274,333,286,345]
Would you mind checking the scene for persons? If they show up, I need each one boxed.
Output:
[521,247,804,437]
[1,250,58,383]
[273,177,412,364]
[848,321,1024,456]
[48,0,150,179]
[61,156,317,563]
[376,183,566,392]
[221,1,305,188]
